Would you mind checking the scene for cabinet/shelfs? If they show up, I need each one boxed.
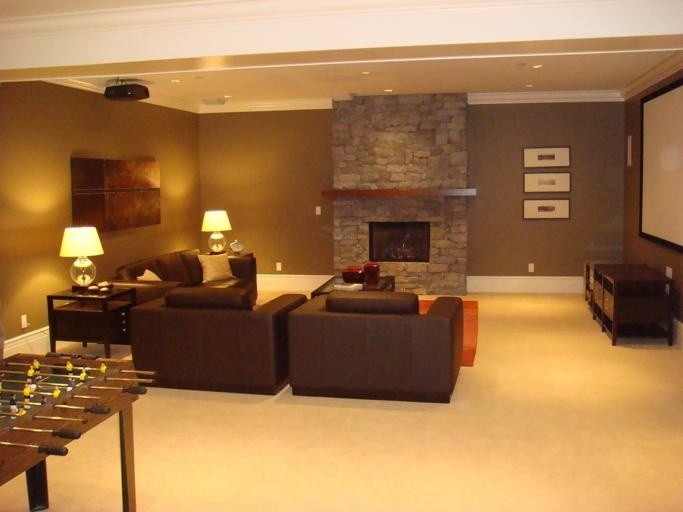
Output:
[582,256,674,348]
[47,288,136,356]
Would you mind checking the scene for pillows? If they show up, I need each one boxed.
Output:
[136,267,163,281]
[197,253,237,284]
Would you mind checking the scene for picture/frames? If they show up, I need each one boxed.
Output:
[522,145,572,222]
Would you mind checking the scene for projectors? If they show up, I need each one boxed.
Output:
[103,84,149,101]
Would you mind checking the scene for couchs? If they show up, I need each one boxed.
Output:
[129,284,308,398]
[115,246,258,308]
[284,290,465,402]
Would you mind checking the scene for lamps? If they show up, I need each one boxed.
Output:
[59,226,104,288]
[199,210,233,252]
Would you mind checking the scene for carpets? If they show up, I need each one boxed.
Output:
[411,297,478,365]
